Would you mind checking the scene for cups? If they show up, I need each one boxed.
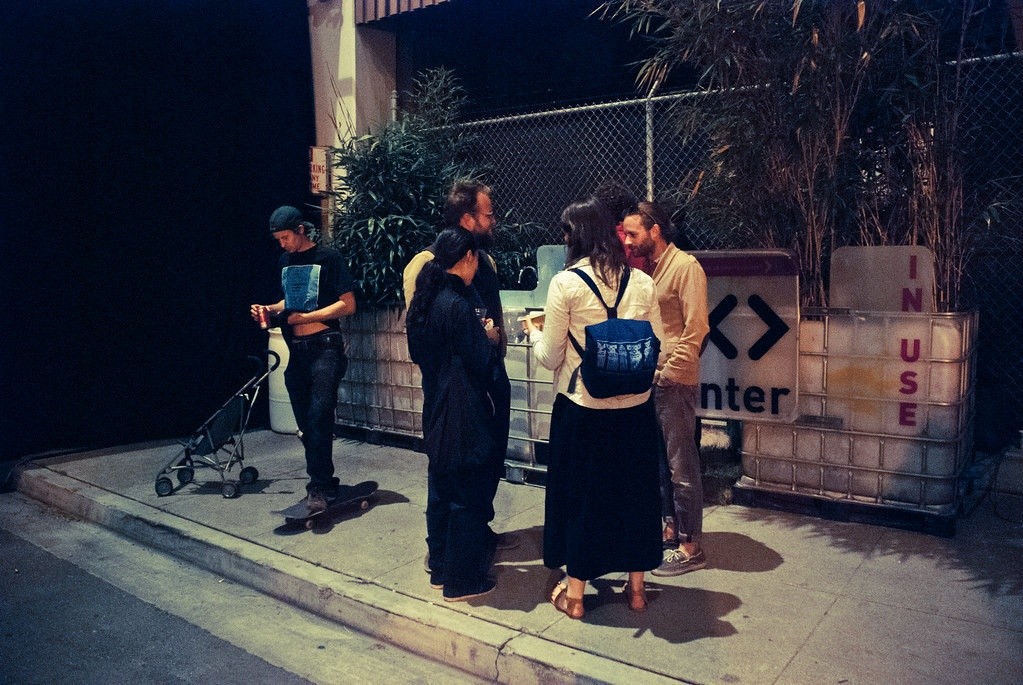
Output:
[474,308,487,326]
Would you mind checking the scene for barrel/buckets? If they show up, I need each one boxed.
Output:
[268,328,301,434]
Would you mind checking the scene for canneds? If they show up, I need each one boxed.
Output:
[257,306,271,329]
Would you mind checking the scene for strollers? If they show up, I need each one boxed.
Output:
[153,349,281,497]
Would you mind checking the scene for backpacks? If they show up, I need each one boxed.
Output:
[567,266,661,399]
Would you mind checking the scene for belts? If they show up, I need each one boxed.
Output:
[291,334,343,352]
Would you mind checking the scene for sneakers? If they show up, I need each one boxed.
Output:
[662,538,681,551]
[651,549,707,577]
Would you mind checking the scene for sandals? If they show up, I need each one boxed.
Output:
[622,582,649,612]
[548,581,585,620]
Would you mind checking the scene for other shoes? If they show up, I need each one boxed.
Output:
[496,532,522,549]
[443,579,497,601]
[307,483,327,510]
[430,572,446,589]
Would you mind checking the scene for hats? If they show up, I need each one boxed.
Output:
[269,206,314,233]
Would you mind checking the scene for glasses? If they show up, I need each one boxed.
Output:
[469,210,494,221]
[621,205,657,224]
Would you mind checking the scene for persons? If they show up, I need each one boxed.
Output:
[622,202,711,576]
[404,179,521,600]
[524,182,679,619]
[251,205,355,509]
